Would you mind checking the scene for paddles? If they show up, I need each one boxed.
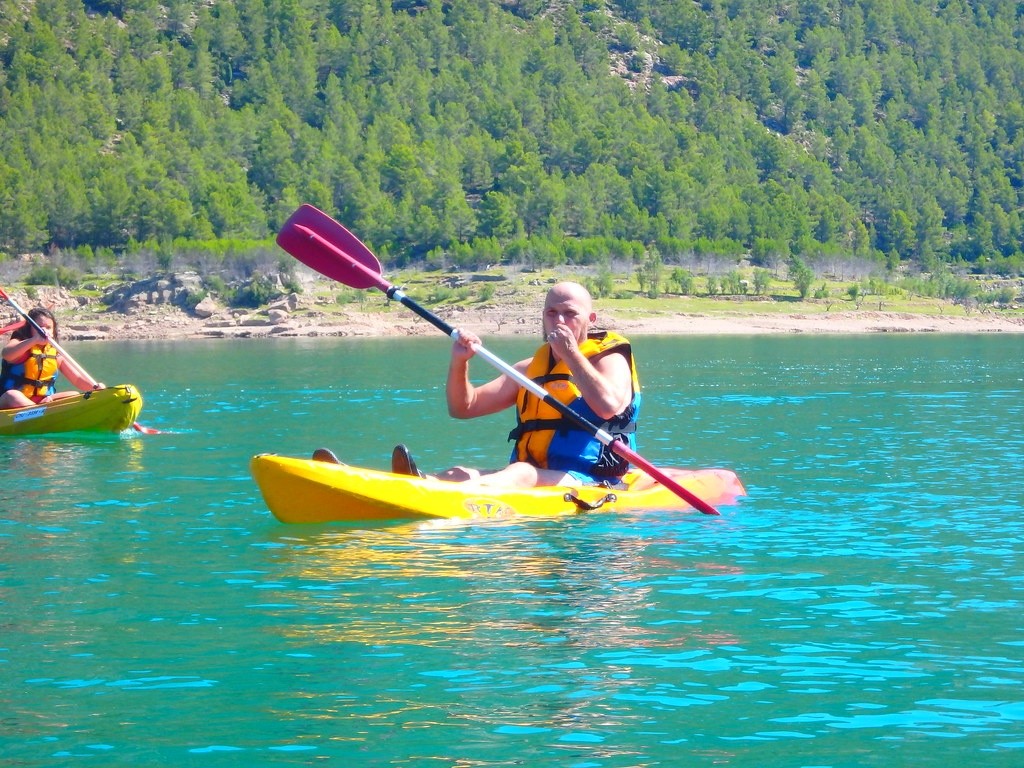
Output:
[0,288,162,435]
[274,200,720,515]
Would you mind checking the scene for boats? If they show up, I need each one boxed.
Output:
[1,386,146,435]
[245,448,747,524]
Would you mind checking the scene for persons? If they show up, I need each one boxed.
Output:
[310,281,641,487]
[0,308,106,410]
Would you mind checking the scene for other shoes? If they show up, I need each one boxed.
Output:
[392,444,428,479]
[312,448,339,463]
